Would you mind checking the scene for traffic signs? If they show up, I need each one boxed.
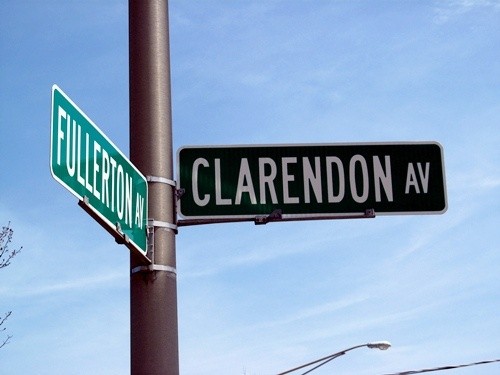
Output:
[51,83,149,256]
[177,141,449,218]
